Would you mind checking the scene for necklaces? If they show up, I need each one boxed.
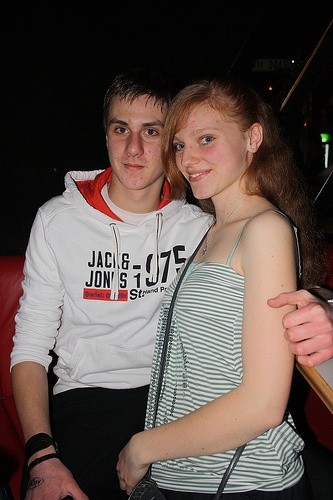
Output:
[201,186,258,256]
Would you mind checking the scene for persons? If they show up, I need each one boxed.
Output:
[114,69,308,499]
[7,61,333,500]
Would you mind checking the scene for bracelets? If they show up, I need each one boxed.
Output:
[26,452,62,471]
[25,432,58,463]
